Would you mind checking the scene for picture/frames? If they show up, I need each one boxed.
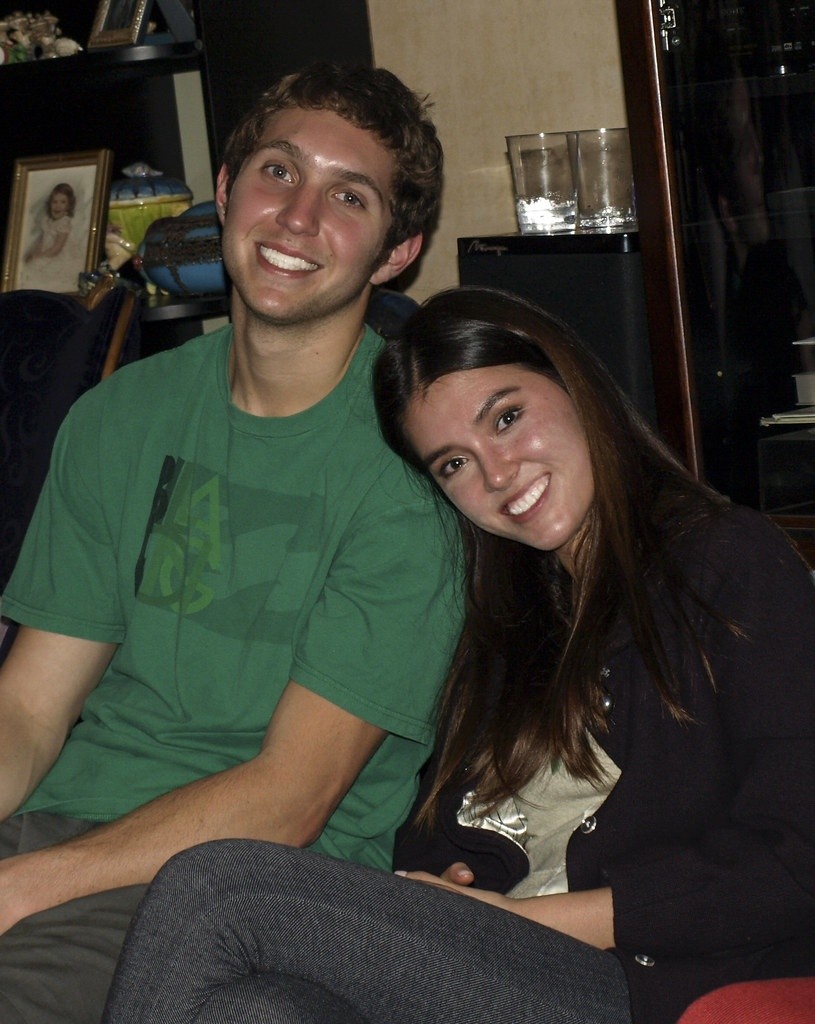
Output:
[0,150,114,298]
[90,0,150,49]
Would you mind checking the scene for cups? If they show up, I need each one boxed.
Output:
[503,132,578,234]
[567,127,638,227]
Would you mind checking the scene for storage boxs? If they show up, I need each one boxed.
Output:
[108,191,191,247]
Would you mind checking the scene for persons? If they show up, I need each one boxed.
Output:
[99,287,815,1024]
[20,184,78,291]
[0,69,476,1024]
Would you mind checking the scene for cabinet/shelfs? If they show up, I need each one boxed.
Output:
[0,1,374,597]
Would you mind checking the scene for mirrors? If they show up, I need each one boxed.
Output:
[610,0,815,548]
